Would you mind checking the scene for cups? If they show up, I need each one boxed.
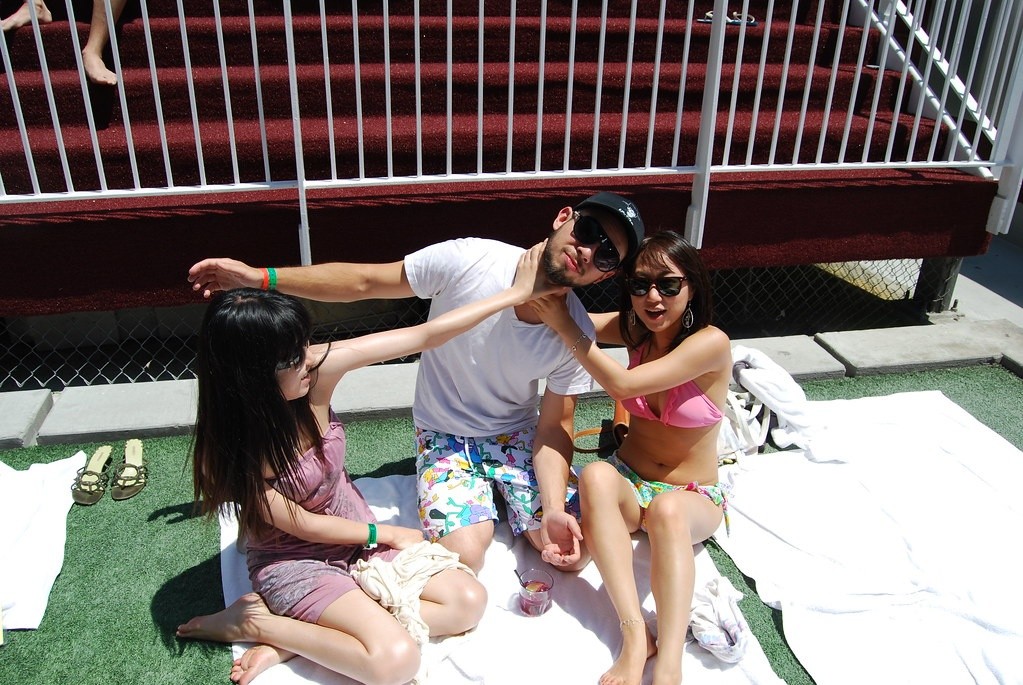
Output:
[517,568,554,617]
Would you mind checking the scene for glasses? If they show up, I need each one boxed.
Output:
[275,339,310,371]
[625,276,691,298]
[573,211,621,272]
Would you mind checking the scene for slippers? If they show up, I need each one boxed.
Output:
[71,445,113,505]
[111,438,148,501]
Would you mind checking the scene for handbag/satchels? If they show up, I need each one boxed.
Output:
[572,400,630,453]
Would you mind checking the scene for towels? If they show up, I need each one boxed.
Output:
[711,389,1023,685]
[219,474,790,685]
[0,450,87,631]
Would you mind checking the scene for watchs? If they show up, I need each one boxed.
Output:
[362,523,378,551]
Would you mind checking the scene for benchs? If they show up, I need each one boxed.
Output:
[0,0,1000,318]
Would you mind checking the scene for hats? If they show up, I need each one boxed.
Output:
[572,192,645,270]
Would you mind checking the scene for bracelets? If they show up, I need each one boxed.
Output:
[187,191,647,572]
[570,333,587,359]
[260,268,277,292]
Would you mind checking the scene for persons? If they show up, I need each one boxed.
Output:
[176,238,571,685]
[527,229,733,685]
[0,0,128,84]
